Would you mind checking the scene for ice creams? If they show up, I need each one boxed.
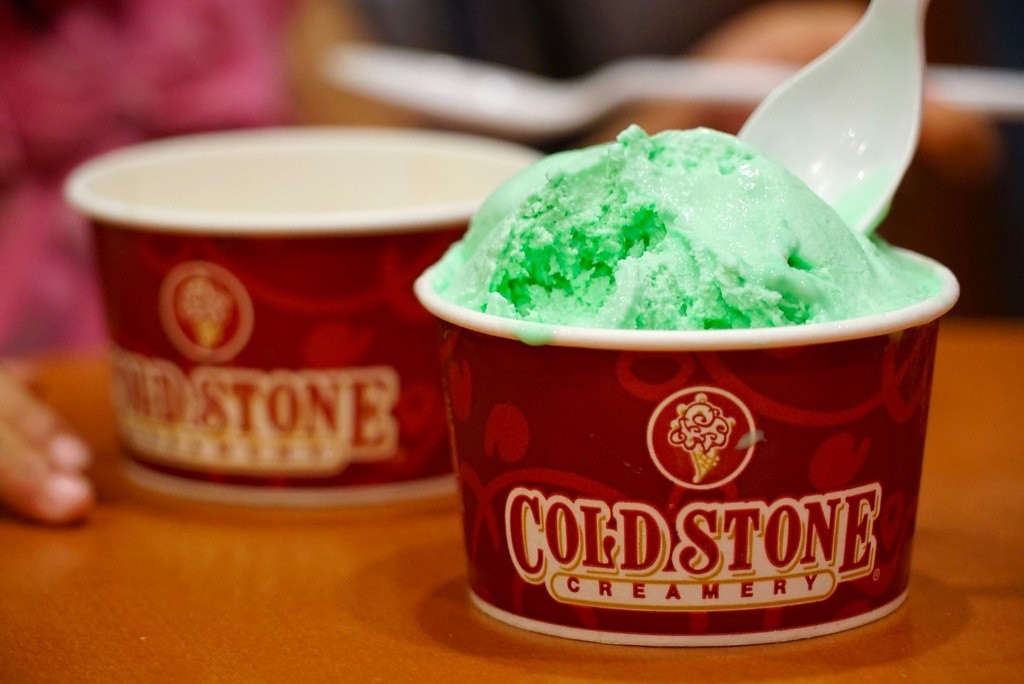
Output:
[410,122,960,646]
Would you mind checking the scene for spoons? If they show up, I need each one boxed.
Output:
[315,42,1024,142]
[733,0,929,239]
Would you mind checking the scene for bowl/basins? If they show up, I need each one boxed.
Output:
[414,243,963,647]
[64,124,548,510]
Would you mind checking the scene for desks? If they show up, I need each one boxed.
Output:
[0,317,1024,684]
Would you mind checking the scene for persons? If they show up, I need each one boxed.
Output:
[582,0,998,200]
[0,370,94,525]
